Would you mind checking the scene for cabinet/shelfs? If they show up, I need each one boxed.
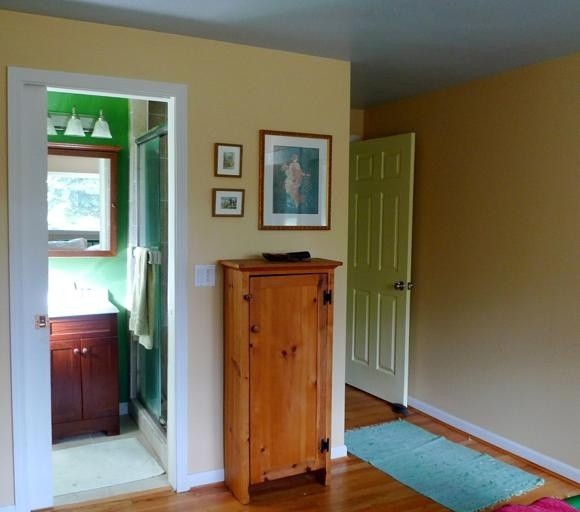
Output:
[50,315,120,443]
[223,259,341,506]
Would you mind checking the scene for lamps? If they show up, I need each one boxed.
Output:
[48,105,113,139]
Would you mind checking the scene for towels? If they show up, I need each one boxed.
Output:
[130,244,157,352]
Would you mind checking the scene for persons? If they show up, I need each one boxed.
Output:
[280,153,310,207]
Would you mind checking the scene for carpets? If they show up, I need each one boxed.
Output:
[51,437,165,497]
[343,419,547,511]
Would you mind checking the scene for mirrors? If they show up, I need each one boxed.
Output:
[47,141,121,257]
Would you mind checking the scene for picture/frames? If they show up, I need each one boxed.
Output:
[257,130,333,229]
[211,140,248,218]
